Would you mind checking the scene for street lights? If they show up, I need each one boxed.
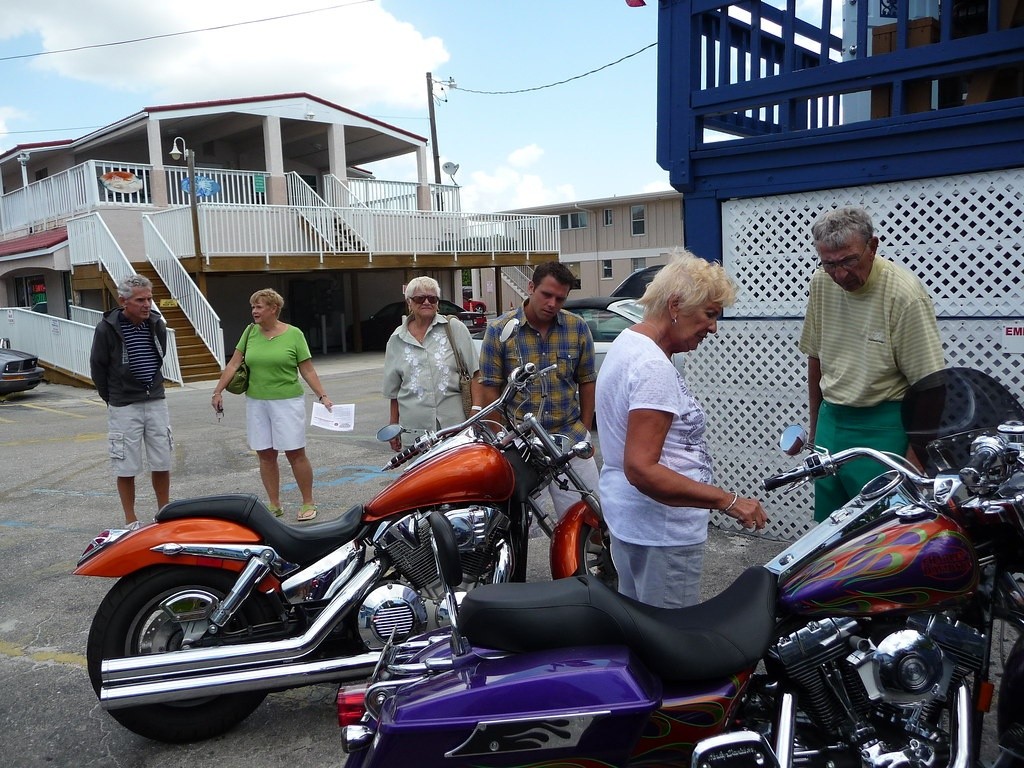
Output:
[169,137,202,290]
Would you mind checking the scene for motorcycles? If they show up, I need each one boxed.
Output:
[71,356,621,741]
[335,368,1023,768]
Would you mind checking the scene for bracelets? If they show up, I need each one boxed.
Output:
[318,395,327,404]
[212,392,221,398]
[723,491,737,512]
[472,406,482,411]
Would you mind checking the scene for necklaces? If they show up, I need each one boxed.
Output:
[414,323,423,342]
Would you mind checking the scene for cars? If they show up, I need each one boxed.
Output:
[608,264,666,298]
[561,297,685,385]
[344,298,488,354]
[463,296,486,314]
[0,348,45,398]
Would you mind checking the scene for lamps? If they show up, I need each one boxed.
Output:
[169,136,188,162]
[442,162,459,186]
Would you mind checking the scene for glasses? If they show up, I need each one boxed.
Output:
[408,295,439,304]
[814,236,870,272]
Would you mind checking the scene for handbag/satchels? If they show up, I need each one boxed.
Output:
[462,374,473,419]
[226,362,250,395]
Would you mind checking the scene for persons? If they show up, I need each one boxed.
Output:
[798,205,946,524]
[382,276,485,467]
[211,289,334,520]
[90,274,174,531]
[594,251,768,609]
[476,260,605,545]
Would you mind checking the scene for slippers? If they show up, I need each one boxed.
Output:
[266,505,282,516]
[297,503,317,520]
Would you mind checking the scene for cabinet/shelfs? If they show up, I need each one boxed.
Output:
[870,16,940,120]
